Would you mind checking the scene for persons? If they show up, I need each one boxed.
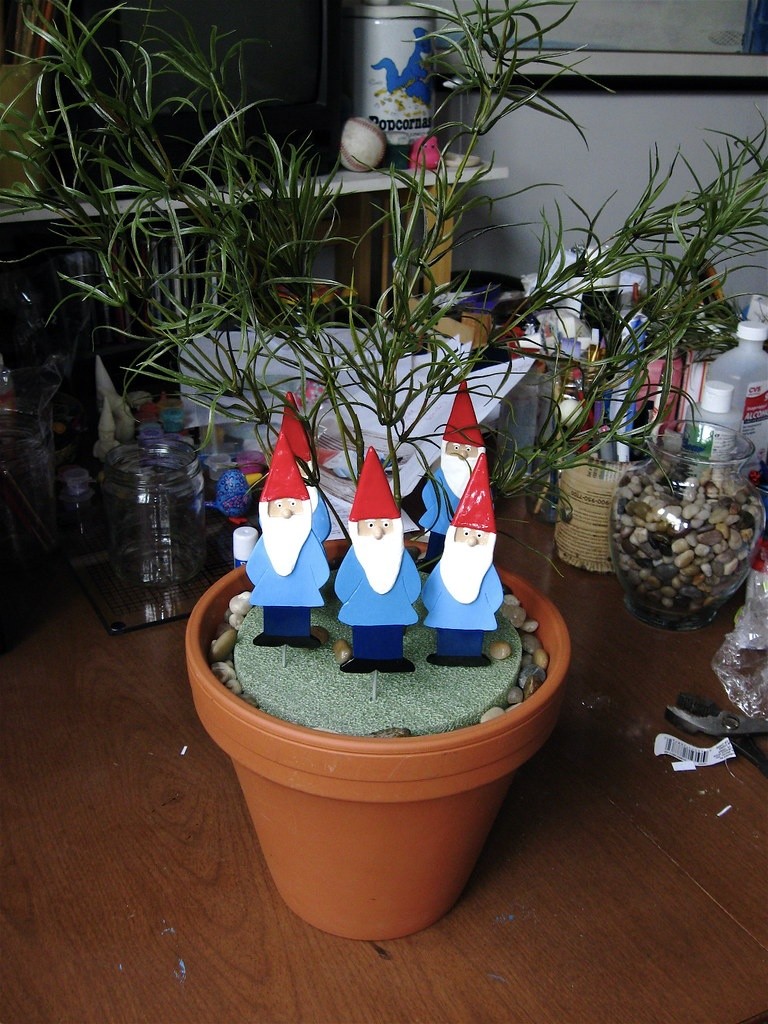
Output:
[334,446,422,674]
[281,393,332,544]
[247,433,330,648]
[420,383,482,575]
[422,454,503,668]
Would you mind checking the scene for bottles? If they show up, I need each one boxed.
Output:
[684,379,744,477]
[60,469,100,538]
[103,436,208,587]
[700,318,768,481]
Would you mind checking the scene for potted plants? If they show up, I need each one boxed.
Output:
[0,0,768,944]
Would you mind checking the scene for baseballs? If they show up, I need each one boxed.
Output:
[340,118,385,171]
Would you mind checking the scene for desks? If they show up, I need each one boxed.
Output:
[0,480,768,1024]
